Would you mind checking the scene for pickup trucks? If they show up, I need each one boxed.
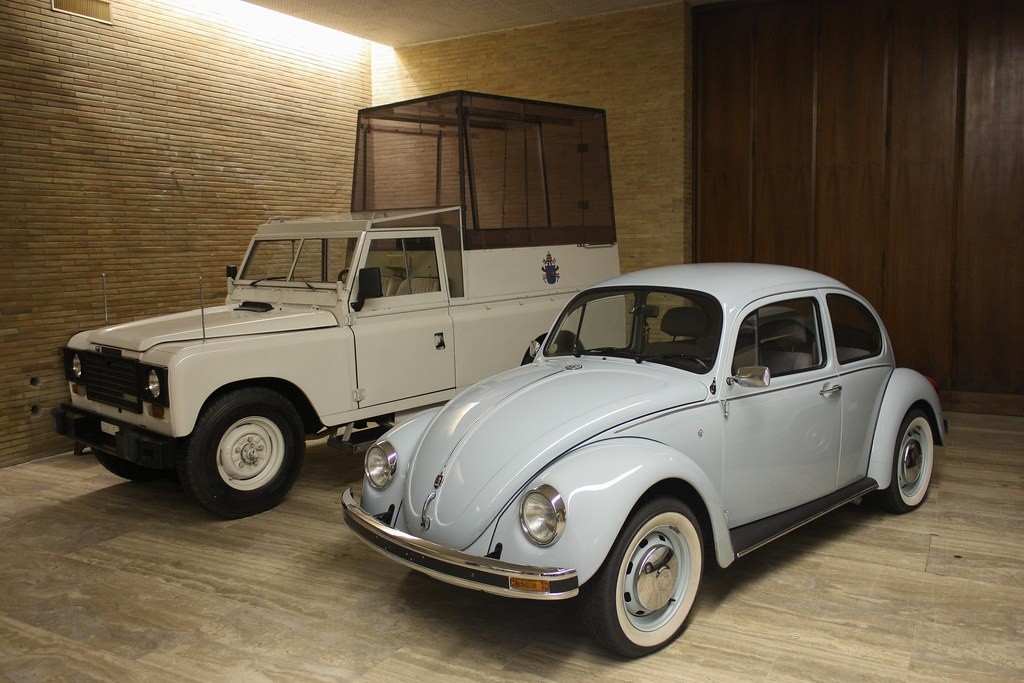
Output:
[46,85,630,522]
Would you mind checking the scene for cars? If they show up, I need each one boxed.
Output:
[339,259,951,661]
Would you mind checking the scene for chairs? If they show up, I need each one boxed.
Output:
[650,306,815,377]
[345,264,440,297]
